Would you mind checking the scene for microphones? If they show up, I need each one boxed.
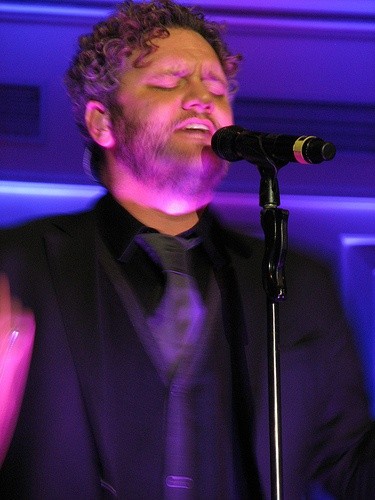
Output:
[211,125,335,164]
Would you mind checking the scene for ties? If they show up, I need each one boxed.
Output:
[135,233,207,371]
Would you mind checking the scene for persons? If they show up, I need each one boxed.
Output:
[0,0,375,500]
